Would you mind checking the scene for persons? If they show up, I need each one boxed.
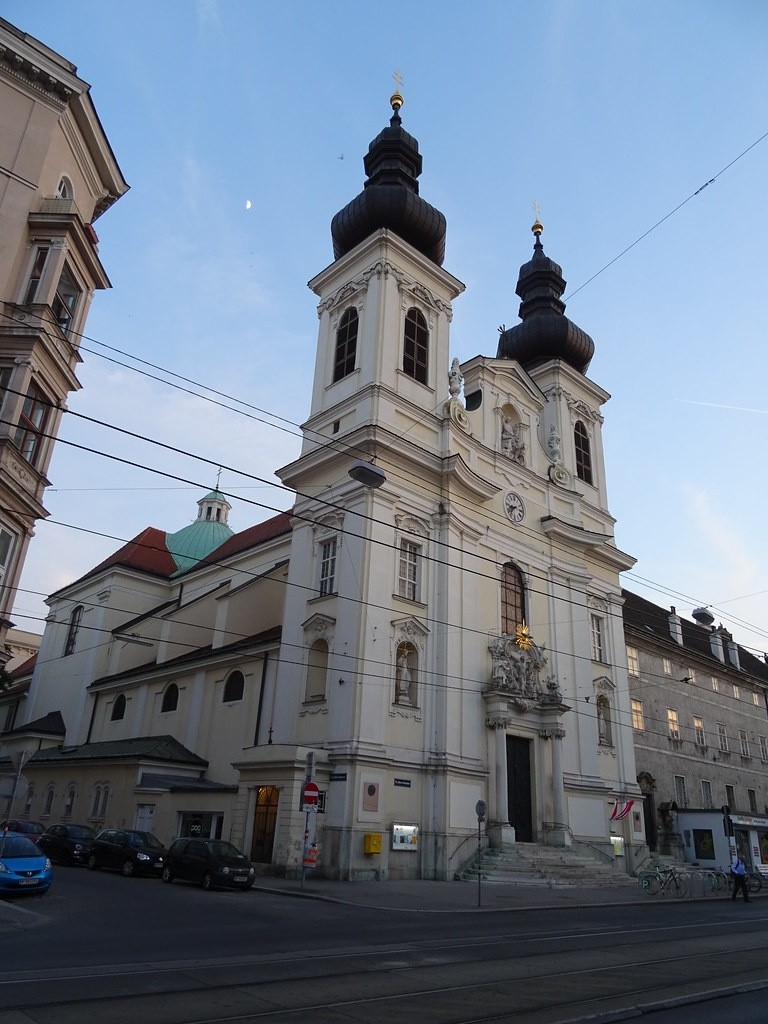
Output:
[730,855,753,902]
[502,415,515,439]
[397,649,411,696]
[597,702,607,737]
[519,653,528,695]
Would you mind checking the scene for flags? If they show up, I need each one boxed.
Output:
[610,798,635,821]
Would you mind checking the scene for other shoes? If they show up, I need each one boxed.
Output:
[731,899,736,902]
[744,899,753,903]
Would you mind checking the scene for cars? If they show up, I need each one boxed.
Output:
[35,823,99,867]
[83,828,168,878]
[162,837,257,891]
[0,818,48,842]
[0,830,54,895]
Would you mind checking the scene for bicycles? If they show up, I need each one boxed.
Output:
[642,863,762,899]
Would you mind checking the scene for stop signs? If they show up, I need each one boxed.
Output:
[303,783,320,804]
